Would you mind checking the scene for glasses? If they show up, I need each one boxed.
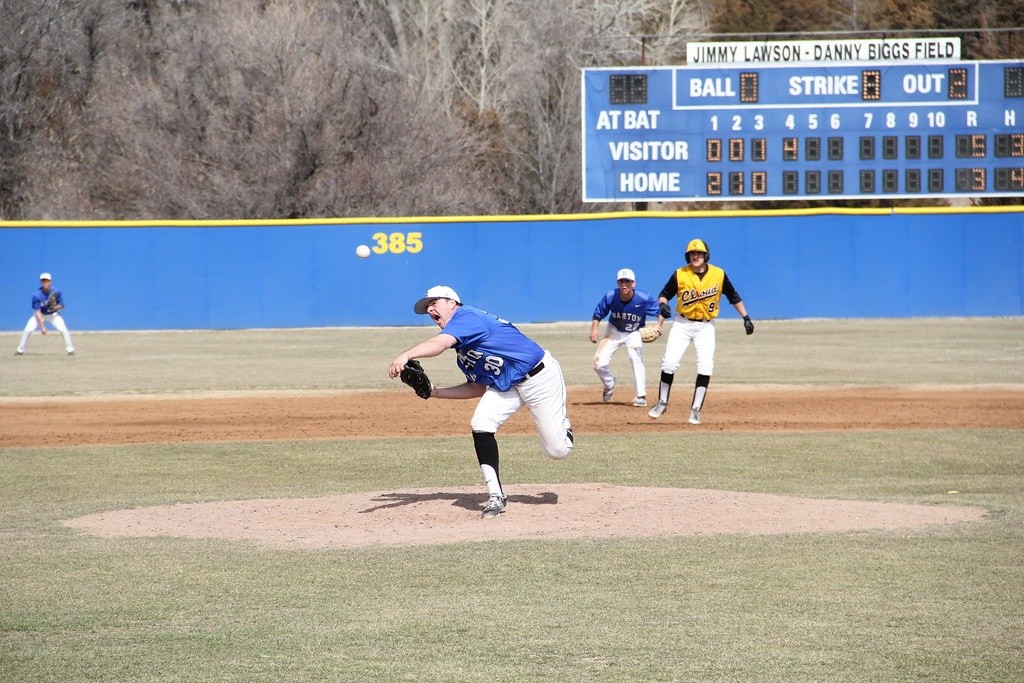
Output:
[618,278,631,283]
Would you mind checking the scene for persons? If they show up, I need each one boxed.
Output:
[15,272,74,356]
[590,268,665,406]
[388,285,573,518]
[648,239,754,424]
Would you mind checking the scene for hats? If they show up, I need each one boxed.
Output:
[616,269,635,282]
[40,272,51,280]
[414,286,460,315]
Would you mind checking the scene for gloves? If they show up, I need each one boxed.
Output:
[657,302,671,318]
[742,314,754,335]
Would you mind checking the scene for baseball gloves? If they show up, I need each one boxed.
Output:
[48,294,57,309]
[638,325,662,343]
[401,360,432,399]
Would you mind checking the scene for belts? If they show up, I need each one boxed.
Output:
[680,313,710,323]
[515,363,544,383]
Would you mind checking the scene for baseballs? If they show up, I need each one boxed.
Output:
[356,245,370,258]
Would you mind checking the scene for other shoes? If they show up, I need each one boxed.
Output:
[69,350,75,354]
[14,352,21,356]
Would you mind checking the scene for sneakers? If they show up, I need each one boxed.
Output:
[648,403,666,418]
[602,378,615,401]
[631,396,647,407]
[687,407,699,424]
[481,492,507,519]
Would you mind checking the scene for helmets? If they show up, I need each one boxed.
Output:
[686,238,707,254]
